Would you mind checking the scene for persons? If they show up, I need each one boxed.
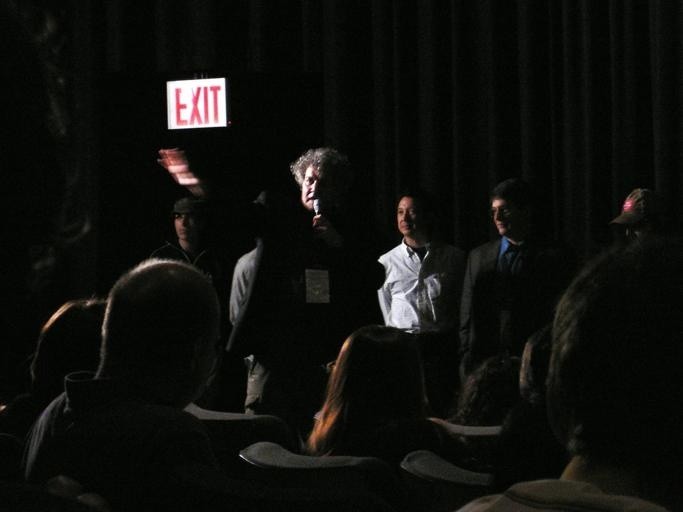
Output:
[2,143,683,510]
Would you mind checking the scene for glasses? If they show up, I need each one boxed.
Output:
[488,206,512,219]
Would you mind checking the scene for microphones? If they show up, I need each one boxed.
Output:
[313,199,324,215]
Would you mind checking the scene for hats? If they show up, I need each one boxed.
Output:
[173,197,206,215]
[610,189,654,224]
